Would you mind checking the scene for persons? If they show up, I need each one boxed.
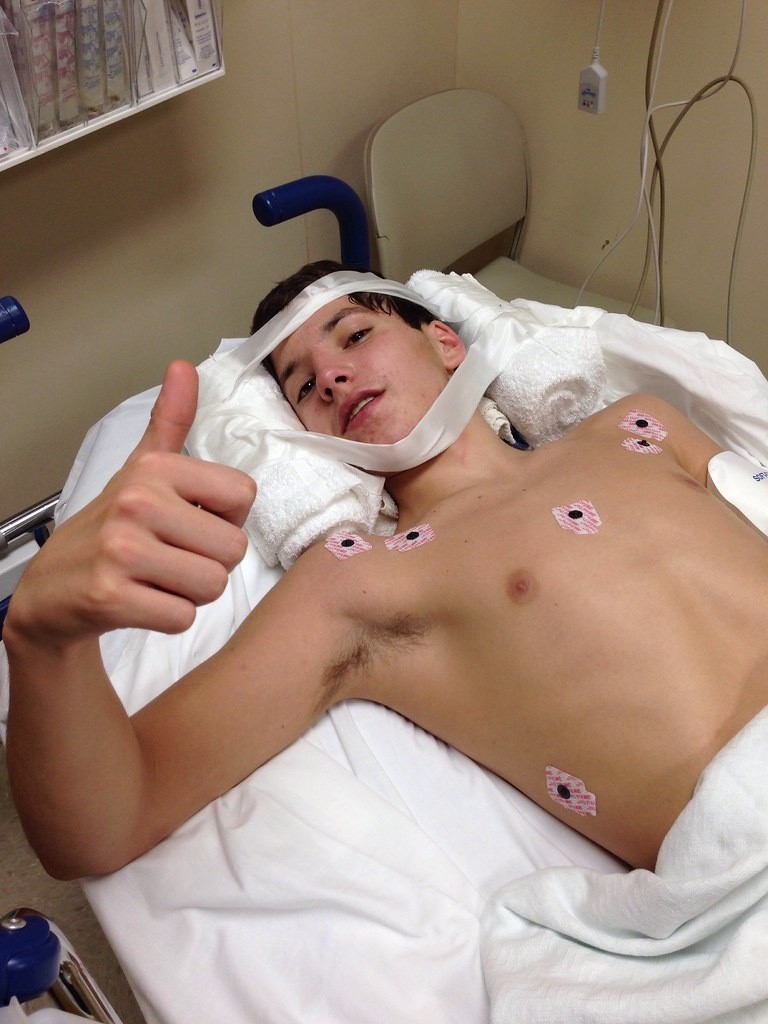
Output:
[0,259,768,903]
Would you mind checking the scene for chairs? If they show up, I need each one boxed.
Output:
[361,87,679,329]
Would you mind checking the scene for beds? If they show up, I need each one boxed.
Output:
[0,173,768,1023]
